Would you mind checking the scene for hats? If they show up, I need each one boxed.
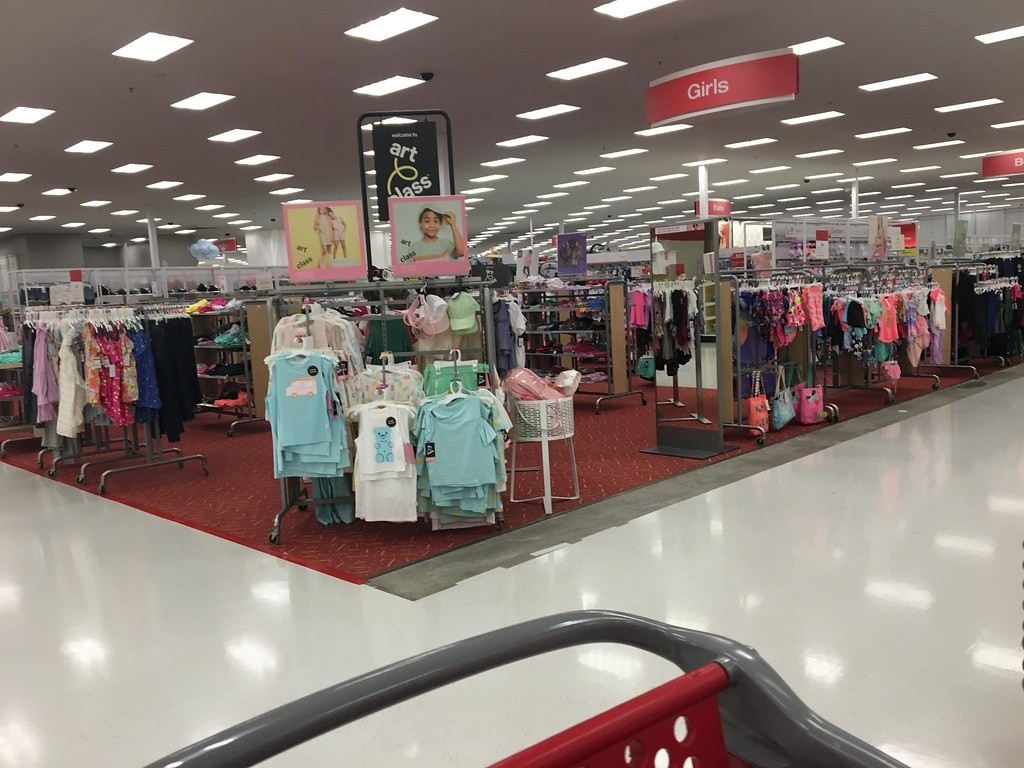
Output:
[417,203,451,225]
[403,294,449,341]
[447,291,480,336]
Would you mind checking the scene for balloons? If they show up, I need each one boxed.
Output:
[190,239,219,262]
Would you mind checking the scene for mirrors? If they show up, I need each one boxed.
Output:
[650,221,721,451]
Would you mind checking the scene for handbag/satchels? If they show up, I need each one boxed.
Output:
[771,366,796,430]
[746,371,769,436]
[787,362,807,420]
[800,363,824,425]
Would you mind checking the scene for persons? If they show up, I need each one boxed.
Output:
[315,208,346,268]
[523,250,532,278]
[408,203,464,263]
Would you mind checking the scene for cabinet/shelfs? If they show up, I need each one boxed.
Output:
[8,265,289,333]
[190,302,279,412]
[509,284,628,384]
[0,362,36,442]
[745,219,900,267]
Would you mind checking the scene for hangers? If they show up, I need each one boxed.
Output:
[653,279,695,298]
[738,272,938,303]
[958,251,1024,295]
[21,303,192,334]
[263,302,493,420]
[632,280,651,294]
